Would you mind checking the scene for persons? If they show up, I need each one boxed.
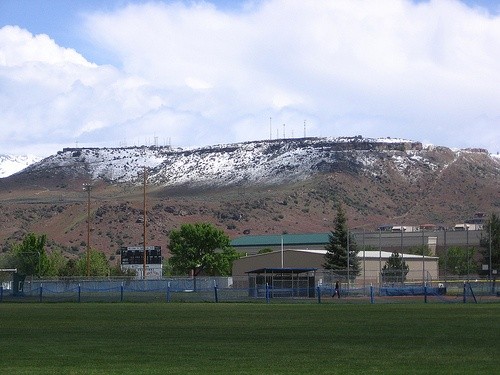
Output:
[332,280,340,299]
